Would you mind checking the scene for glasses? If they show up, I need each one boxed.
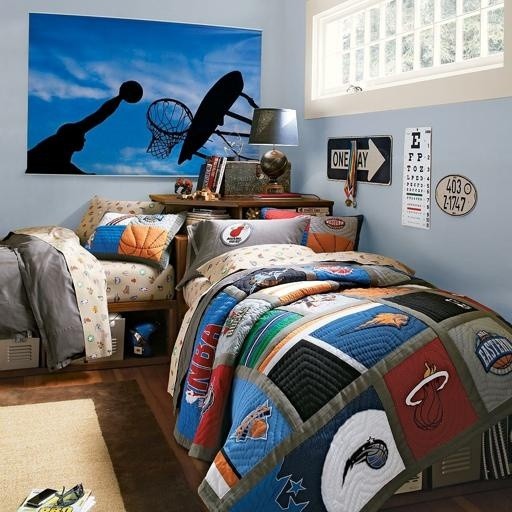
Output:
[56,483,84,508]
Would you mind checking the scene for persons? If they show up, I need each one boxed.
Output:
[27,80,128,175]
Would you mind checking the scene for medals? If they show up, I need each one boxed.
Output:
[345,199,352,207]
[352,201,358,208]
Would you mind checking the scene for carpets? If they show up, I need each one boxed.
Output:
[1,379,202,511]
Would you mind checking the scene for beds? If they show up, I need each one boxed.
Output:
[0,244,178,363]
[172,235,511,511]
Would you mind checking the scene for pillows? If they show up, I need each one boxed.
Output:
[175,208,364,291]
[76,195,185,273]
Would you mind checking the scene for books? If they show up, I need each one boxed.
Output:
[185,207,232,227]
[16,487,93,512]
[258,193,301,198]
[197,155,228,194]
[297,206,329,213]
[257,197,303,200]
[302,213,329,216]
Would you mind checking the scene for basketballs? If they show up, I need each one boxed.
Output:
[119,224,166,261]
[482,335,512,368]
[368,444,387,467]
[119,80,143,103]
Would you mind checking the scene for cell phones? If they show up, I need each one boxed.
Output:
[27,488,57,507]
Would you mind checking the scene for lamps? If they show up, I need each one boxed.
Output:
[248,108,301,196]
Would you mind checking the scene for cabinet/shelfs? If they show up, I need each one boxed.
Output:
[149,193,333,237]
